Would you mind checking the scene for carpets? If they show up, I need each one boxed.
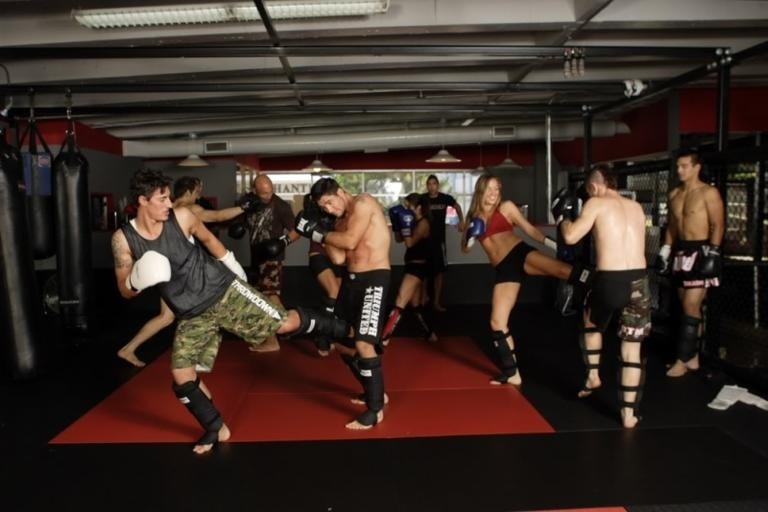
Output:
[525,506,628,512]
[48,335,556,444]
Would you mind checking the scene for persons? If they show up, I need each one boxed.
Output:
[460,174,593,387]
[111,170,355,456]
[550,164,652,430]
[655,148,726,377]
[293,178,392,432]
[229,177,351,358]
[380,175,461,352]
[112,177,250,367]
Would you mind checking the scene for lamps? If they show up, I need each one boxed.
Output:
[177,155,210,167]
[301,160,333,171]
[70,0,389,32]
[425,150,462,163]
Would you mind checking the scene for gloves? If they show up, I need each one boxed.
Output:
[654,255,669,273]
[389,205,415,237]
[550,187,578,223]
[554,243,586,262]
[703,246,721,278]
[125,251,172,293]
[465,218,485,247]
[220,193,336,281]
